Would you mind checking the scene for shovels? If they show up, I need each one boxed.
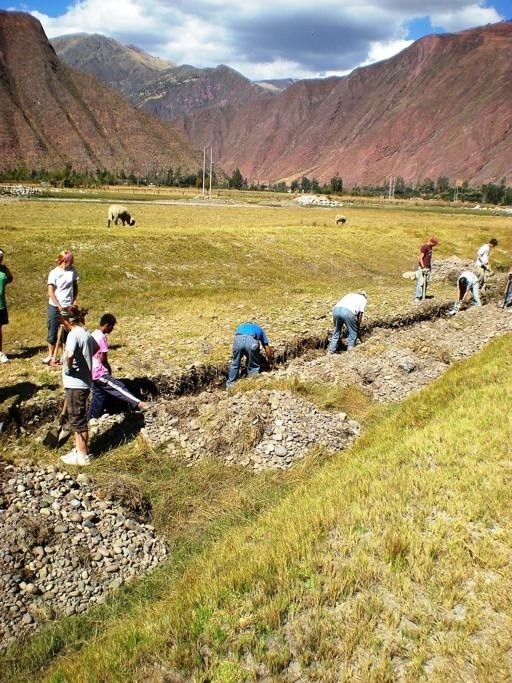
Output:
[42,399,67,451]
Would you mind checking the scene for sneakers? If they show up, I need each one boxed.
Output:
[60,451,90,466]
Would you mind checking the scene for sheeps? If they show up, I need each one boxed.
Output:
[107,204,135,228]
[336,214,347,225]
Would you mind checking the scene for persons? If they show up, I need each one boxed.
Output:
[0,249,13,363]
[413,234,438,301]
[472,238,498,292]
[325,288,368,356]
[87,314,155,420]
[41,248,80,365]
[448,270,482,315]
[225,322,275,392]
[56,306,101,465]
